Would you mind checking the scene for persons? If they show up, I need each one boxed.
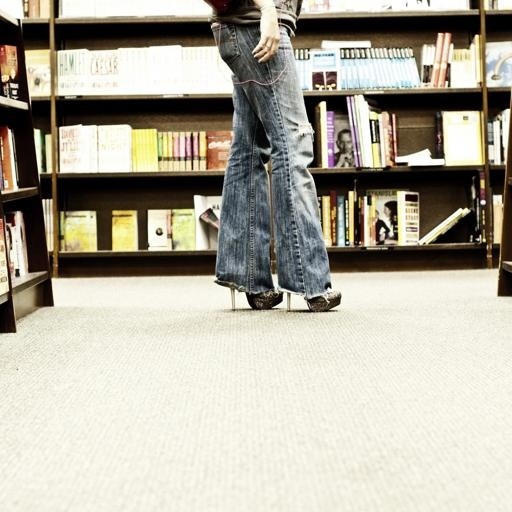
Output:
[205,1,342,313]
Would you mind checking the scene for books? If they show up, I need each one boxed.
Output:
[25,44,236,93]
[33,124,233,172]
[42,196,222,252]
[314,94,511,165]
[299,0,512,16]
[317,172,504,247]
[293,32,512,91]
[0,44,31,297]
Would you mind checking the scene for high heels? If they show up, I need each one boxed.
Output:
[287,285,341,312]
[229,281,283,310]
